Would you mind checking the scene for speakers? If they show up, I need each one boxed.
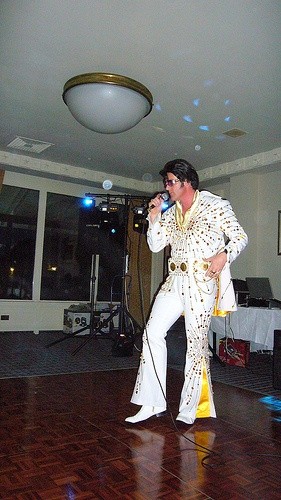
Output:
[165,330,187,365]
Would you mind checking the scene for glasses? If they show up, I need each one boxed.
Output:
[164,179,182,186]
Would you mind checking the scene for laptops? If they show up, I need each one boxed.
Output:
[245,277,281,305]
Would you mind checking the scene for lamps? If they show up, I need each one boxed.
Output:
[61,72,154,135]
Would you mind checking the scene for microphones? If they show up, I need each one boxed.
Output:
[147,193,169,211]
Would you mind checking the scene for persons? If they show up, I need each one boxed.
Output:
[125,158,249,424]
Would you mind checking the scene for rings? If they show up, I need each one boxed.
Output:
[211,271,215,274]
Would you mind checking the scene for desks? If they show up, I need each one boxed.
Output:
[208,303,281,389]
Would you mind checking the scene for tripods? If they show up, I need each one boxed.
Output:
[45,193,153,357]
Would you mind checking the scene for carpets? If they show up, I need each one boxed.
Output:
[0,331,281,399]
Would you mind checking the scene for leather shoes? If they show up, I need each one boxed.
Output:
[125,404,167,423]
[176,412,194,425]
